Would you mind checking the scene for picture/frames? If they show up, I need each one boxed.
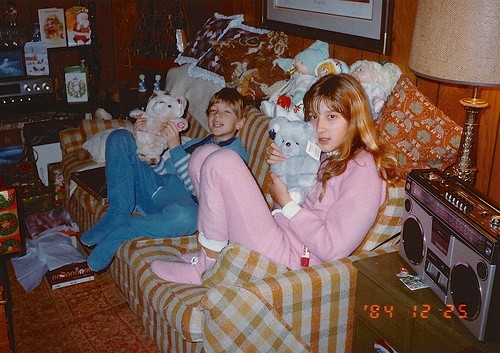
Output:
[259,0,395,57]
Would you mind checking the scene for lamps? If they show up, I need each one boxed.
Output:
[407,0,500,186]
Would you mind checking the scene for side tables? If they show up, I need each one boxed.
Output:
[350,251,500,353]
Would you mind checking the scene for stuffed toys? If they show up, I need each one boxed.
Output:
[305,58,349,96]
[350,61,402,121]
[127,92,190,164]
[267,116,320,210]
[261,40,329,119]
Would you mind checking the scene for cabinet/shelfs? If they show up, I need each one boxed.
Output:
[0,0,106,124]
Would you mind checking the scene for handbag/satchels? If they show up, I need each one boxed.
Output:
[1,144,27,164]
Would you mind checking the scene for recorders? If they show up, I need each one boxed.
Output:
[398,169,500,343]
[0,75,55,117]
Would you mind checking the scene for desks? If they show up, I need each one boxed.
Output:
[125,53,179,99]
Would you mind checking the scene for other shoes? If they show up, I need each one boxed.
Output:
[87,224,132,272]
[150,247,217,285]
[80,209,130,246]
[180,252,200,262]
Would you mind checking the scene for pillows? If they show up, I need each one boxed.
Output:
[188,20,271,86]
[79,118,134,160]
[201,240,293,291]
[373,74,465,179]
[173,12,246,66]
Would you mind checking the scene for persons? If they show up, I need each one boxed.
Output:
[150,73,386,285]
[79,87,249,273]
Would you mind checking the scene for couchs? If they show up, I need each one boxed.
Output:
[56,62,409,353]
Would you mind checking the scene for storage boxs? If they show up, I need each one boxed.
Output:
[0,186,27,261]
[48,161,65,212]
